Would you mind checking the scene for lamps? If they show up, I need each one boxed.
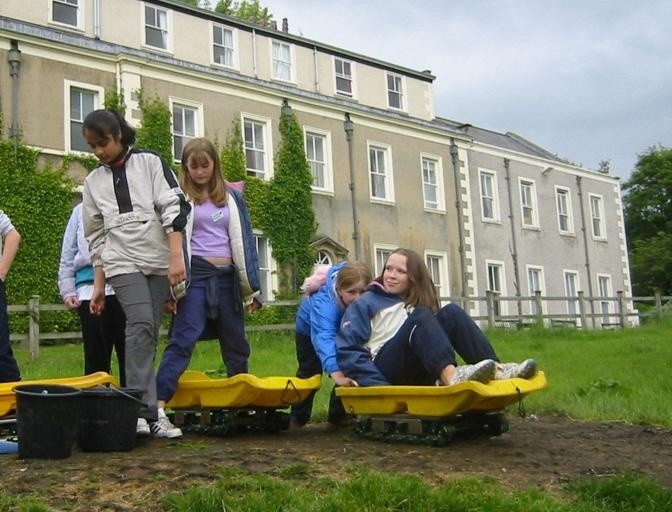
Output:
[541,165,554,176]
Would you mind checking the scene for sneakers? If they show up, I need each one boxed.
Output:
[151,417,183,438]
[136,418,151,435]
[495,359,536,378]
[450,358,496,384]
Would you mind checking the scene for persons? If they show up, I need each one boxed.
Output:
[56,198,128,388]
[79,108,193,439]
[144,137,264,440]
[285,257,374,435]
[333,246,540,387]
[0,206,20,429]
